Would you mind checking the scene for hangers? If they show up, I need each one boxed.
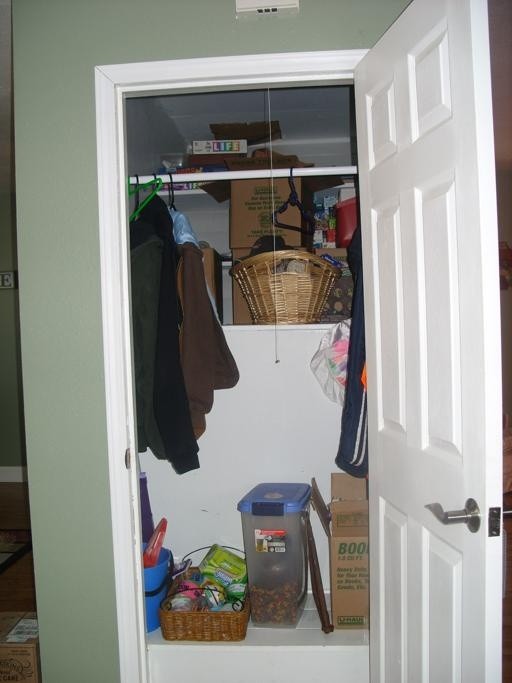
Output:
[129,174,178,220]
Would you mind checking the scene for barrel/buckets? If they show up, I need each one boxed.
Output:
[140,544,174,633]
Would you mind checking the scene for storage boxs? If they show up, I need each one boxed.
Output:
[201,178,349,325]
[0,612,40,683]
[312,472,370,629]
[238,483,312,628]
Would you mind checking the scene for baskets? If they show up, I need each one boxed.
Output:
[159,567,251,641]
[228,249,342,325]
